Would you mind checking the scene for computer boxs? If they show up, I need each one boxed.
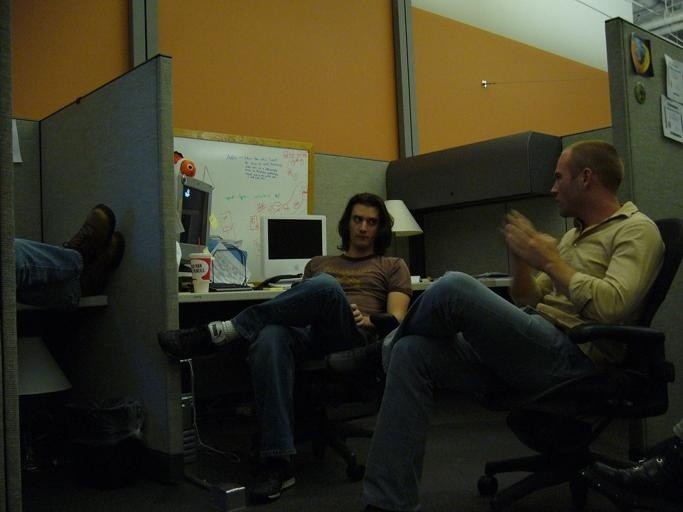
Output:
[179,359,202,463]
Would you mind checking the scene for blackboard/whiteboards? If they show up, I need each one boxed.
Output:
[174,130,314,282]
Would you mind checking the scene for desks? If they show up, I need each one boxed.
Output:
[176,275,515,304]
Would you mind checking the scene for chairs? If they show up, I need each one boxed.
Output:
[294,312,400,481]
[478,216,683,512]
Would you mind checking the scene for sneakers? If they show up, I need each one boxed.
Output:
[576,443,682,508]
[63,205,116,259]
[157,320,228,371]
[76,232,126,292]
[324,343,384,375]
[246,460,297,501]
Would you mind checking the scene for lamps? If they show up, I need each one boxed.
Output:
[18,338,71,473]
[383,200,422,258]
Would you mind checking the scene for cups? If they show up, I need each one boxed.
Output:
[189,252,212,293]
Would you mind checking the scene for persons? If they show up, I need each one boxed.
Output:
[362,139,665,511]
[14,200,126,311]
[585,414,683,512]
[157,192,414,504]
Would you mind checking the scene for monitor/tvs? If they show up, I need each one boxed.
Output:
[177,173,212,277]
[260,214,328,283]
